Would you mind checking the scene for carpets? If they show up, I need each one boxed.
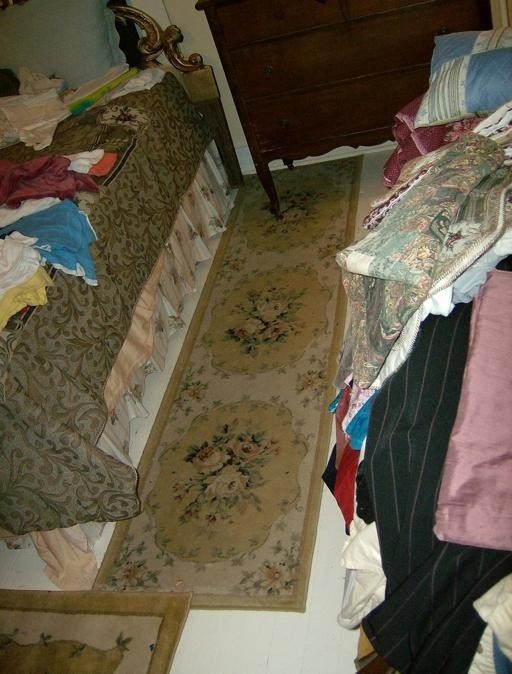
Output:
[86,154,364,611]
[0,591,192,668]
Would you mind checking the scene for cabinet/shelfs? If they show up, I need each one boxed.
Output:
[196,0,499,214]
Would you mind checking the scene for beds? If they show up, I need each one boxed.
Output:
[0,5,237,573]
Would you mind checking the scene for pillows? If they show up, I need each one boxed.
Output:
[0,0,125,89]
